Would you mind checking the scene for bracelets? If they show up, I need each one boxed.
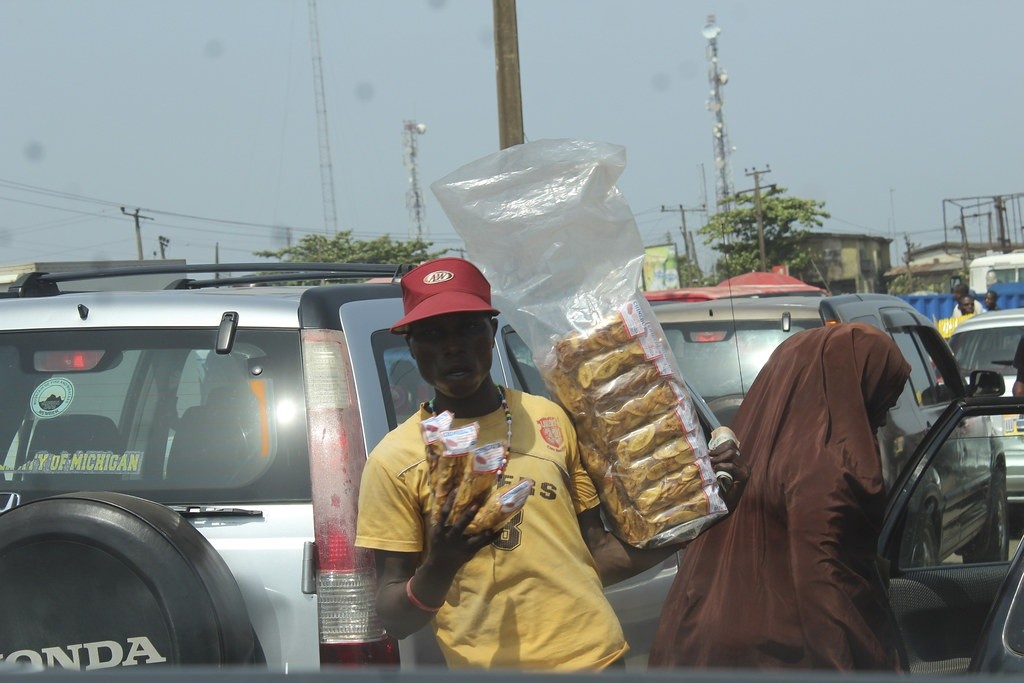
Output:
[407,575,446,614]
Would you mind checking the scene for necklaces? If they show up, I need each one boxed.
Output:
[429,383,511,483]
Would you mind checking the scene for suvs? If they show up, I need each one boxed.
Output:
[2,260,729,680]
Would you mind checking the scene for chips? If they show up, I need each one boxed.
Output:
[420,311,715,547]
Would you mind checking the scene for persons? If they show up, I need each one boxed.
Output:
[958,297,976,318]
[949,281,985,320]
[984,291,1000,311]
[347,257,750,675]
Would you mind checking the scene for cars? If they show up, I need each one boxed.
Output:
[870,391,1024,679]
[937,307,1024,505]
[655,293,1010,565]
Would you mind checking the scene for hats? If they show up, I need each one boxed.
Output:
[390,257,502,335]
